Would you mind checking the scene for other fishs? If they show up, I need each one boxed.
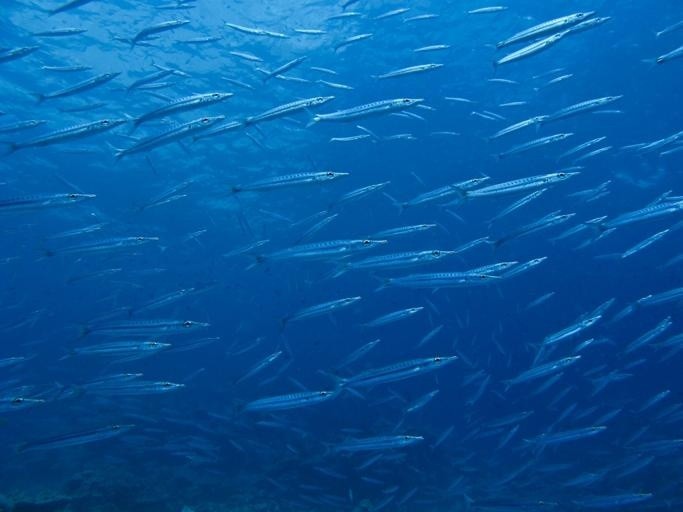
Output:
[0,0,683,512]
